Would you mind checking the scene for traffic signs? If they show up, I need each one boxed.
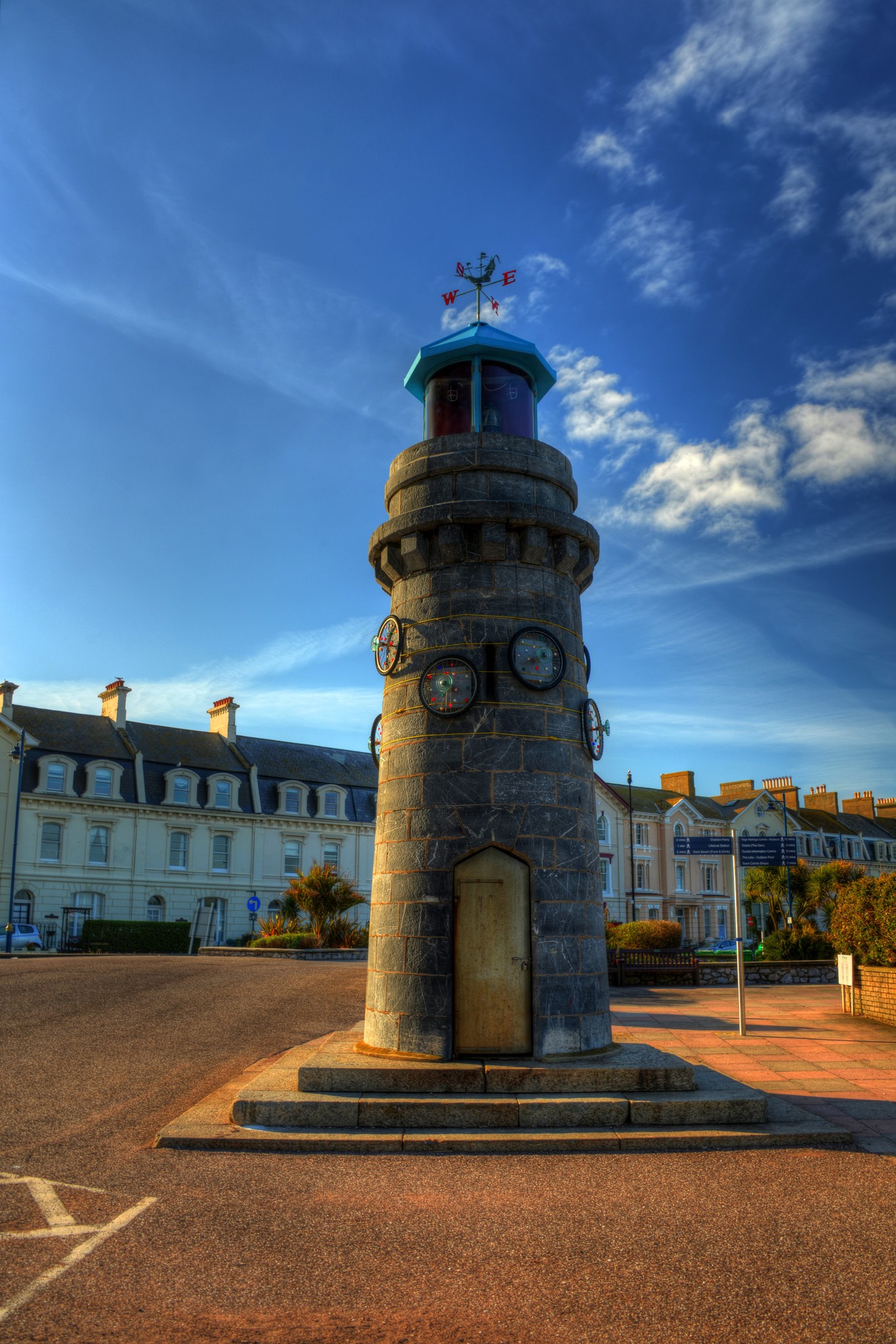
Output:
[247,896,261,912]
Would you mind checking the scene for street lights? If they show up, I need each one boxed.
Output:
[5,727,27,953]
[824,831,844,860]
[763,794,792,932]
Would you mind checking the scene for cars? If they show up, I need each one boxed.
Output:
[700,936,726,947]
[696,940,737,954]
[0,922,44,952]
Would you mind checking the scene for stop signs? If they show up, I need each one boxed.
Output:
[748,917,757,927]
